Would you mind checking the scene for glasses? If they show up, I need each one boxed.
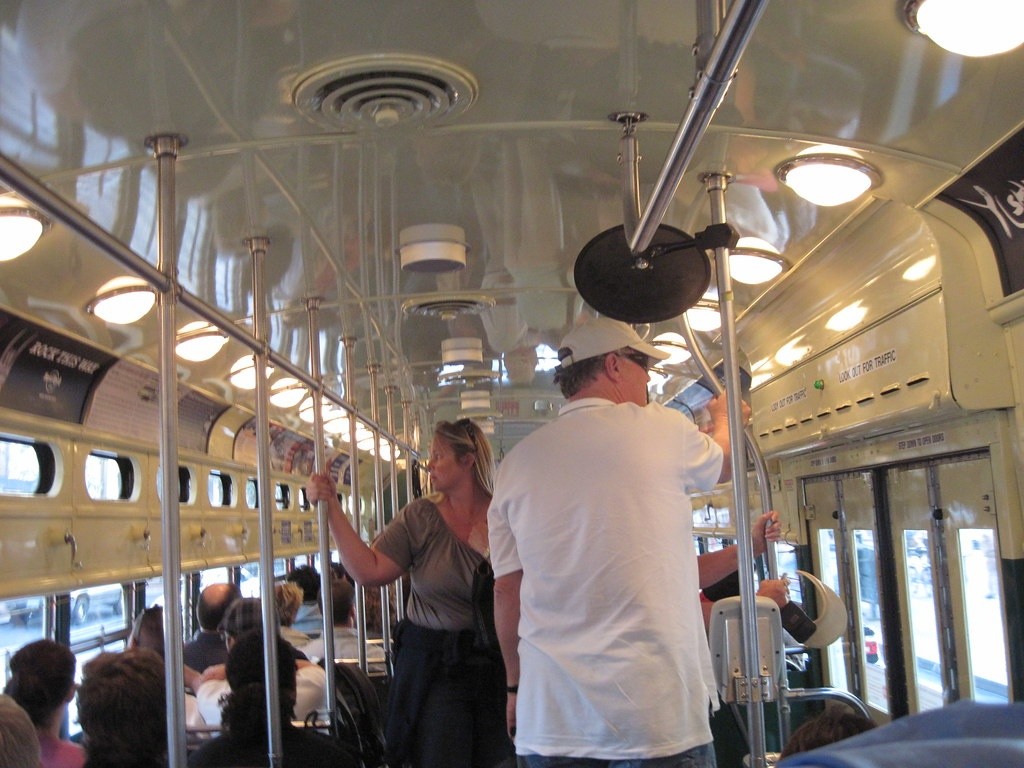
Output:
[601,351,648,370]
[459,417,478,449]
[137,605,163,639]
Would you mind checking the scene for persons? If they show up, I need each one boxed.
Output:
[305,418,495,768]
[1,694,42,768]
[778,709,878,762]
[486,318,750,768]
[697,511,792,635]
[126,561,397,749]
[11,640,88,768]
[186,629,355,768]
[76,649,167,767]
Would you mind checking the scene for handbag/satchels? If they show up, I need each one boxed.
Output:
[472,557,501,657]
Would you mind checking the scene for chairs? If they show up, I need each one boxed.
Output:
[334,658,386,696]
[186,727,229,758]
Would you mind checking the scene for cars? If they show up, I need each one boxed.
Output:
[11,582,124,628]
[199,564,261,599]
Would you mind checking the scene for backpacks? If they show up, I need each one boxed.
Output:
[330,661,388,768]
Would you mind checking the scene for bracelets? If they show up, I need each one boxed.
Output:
[507,684,518,694]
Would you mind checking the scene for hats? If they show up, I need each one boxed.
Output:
[780,571,848,648]
[558,317,670,370]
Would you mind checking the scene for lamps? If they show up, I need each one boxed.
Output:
[772,153,885,208]
[175,331,229,363]
[0,206,52,262]
[713,247,792,285]
[269,384,307,408]
[85,285,158,325]
[298,403,332,423]
[222,364,275,390]
[903,0,1024,59]
[650,341,692,365]
[686,300,721,332]
[648,367,668,386]
